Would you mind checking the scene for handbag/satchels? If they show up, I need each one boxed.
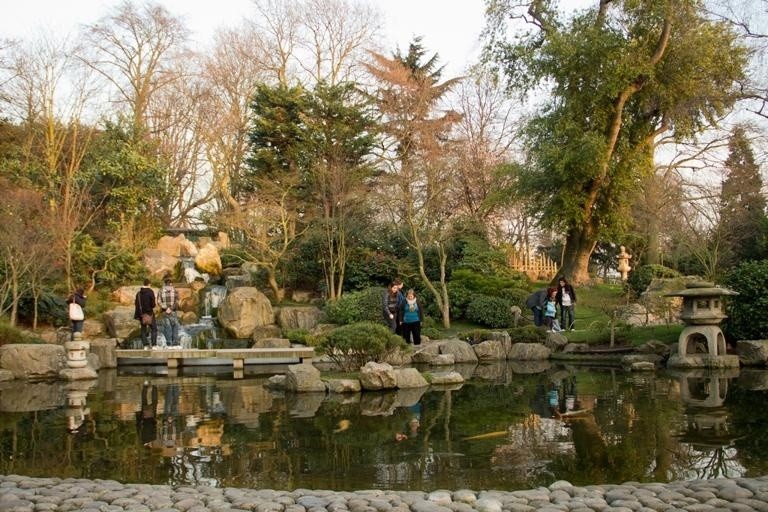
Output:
[68,302,84,321]
[141,313,153,325]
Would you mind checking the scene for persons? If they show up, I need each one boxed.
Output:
[382,282,400,333]
[160,383,180,466]
[66,288,87,341]
[156,279,183,350]
[398,287,424,345]
[557,278,577,332]
[534,375,577,412]
[134,381,159,449]
[526,287,559,326]
[133,279,159,350]
[393,277,404,336]
[544,296,557,333]
[395,397,422,442]
